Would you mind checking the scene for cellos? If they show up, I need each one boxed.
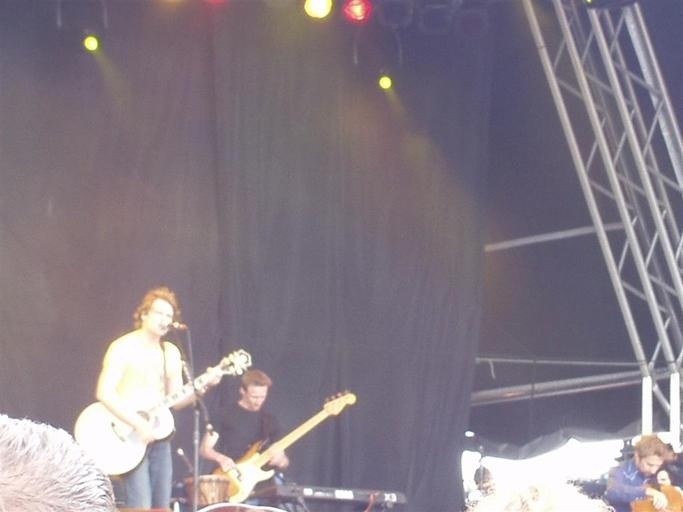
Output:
[630,474,683,512]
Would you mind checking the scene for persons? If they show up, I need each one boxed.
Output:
[662,443,682,490]
[602,434,667,512]
[95,285,222,512]
[200,368,289,508]
[1,414,117,512]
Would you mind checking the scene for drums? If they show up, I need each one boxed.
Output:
[185,476,230,512]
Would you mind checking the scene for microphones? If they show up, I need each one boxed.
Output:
[163,320,185,330]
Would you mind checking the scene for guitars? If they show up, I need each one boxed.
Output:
[212,391,356,505]
[74,348,252,481]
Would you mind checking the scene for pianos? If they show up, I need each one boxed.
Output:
[250,486,407,505]
